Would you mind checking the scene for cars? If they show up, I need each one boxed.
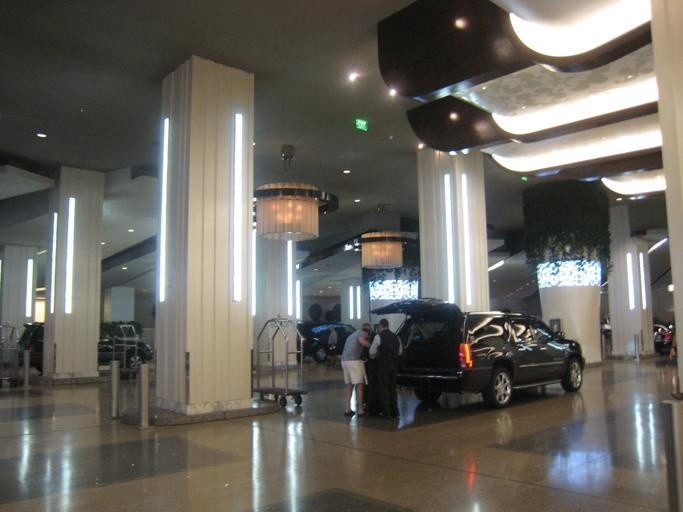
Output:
[297,321,357,363]
[653,323,675,358]
[369,297,585,409]
[15,322,154,375]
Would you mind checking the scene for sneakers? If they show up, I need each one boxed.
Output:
[344,411,355,417]
[357,411,368,417]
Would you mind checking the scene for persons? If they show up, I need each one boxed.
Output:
[325,326,339,367]
[368,318,402,419]
[340,323,371,419]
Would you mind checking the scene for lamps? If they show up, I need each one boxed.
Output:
[483,112,662,182]
[254,144,339,241]
[596,166,668,198]
[463,38,659,146]
[359,206,419,271]
[463,0,653,87]
